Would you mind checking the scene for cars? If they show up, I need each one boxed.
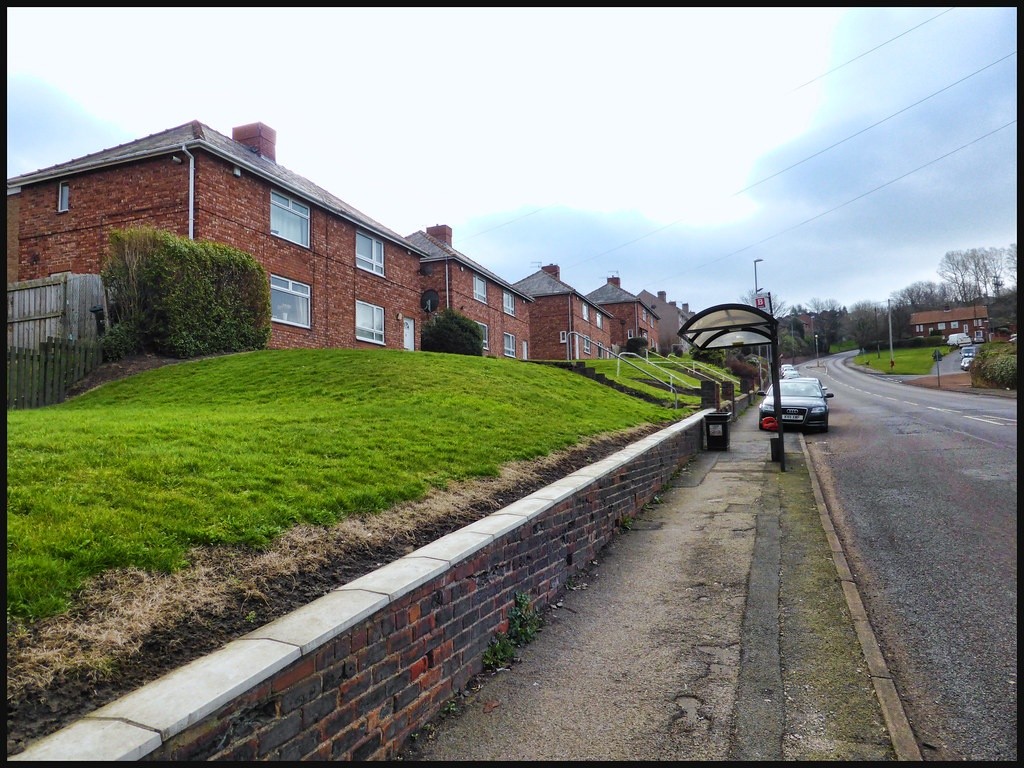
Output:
[757,380,834,432]
[780,364,800,379]
[948,333,985,371]
[791,377,828,396]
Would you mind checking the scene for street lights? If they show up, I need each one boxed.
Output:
[815,335,820,367]
[753,258,764,395]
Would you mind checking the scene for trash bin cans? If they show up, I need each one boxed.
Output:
[704,411,732,451]
